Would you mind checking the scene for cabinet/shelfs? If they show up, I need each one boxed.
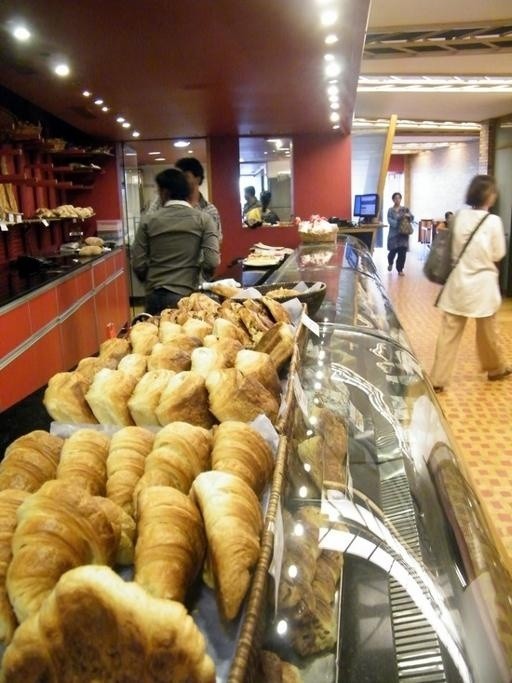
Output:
[92,249,128,351]
[0,134,115,225]
[0,287,65,414]
[56,268,100,371]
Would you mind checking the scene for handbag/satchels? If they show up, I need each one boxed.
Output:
[423,227,452,283]
[398,216,414,235]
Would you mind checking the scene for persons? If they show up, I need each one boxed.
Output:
[242,185,261,221]
[142,155,222,254]
[131,167,221,315]
[385,190,414,276]
[427,175,511,394]
[433,209,452,231]
[260,193,280,224]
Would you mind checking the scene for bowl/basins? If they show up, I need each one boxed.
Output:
[251,279,327,317]
[53,166,100,186]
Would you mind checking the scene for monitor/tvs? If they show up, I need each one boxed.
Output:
[360,194,378,224]
[354,195,360,216]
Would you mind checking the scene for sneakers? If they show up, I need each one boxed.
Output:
[432,382,446,394]
[486,367,510,380]
[398,271,405,276]
[387,264,393,271]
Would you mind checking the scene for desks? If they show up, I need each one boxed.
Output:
[337,224,388,256]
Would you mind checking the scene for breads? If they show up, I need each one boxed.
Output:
[42,291,295,422]
[78,237,104,256]
[282,403,348,498]
[1,419,275,683]
[270,504,344,658]
[258,650,302,683]
